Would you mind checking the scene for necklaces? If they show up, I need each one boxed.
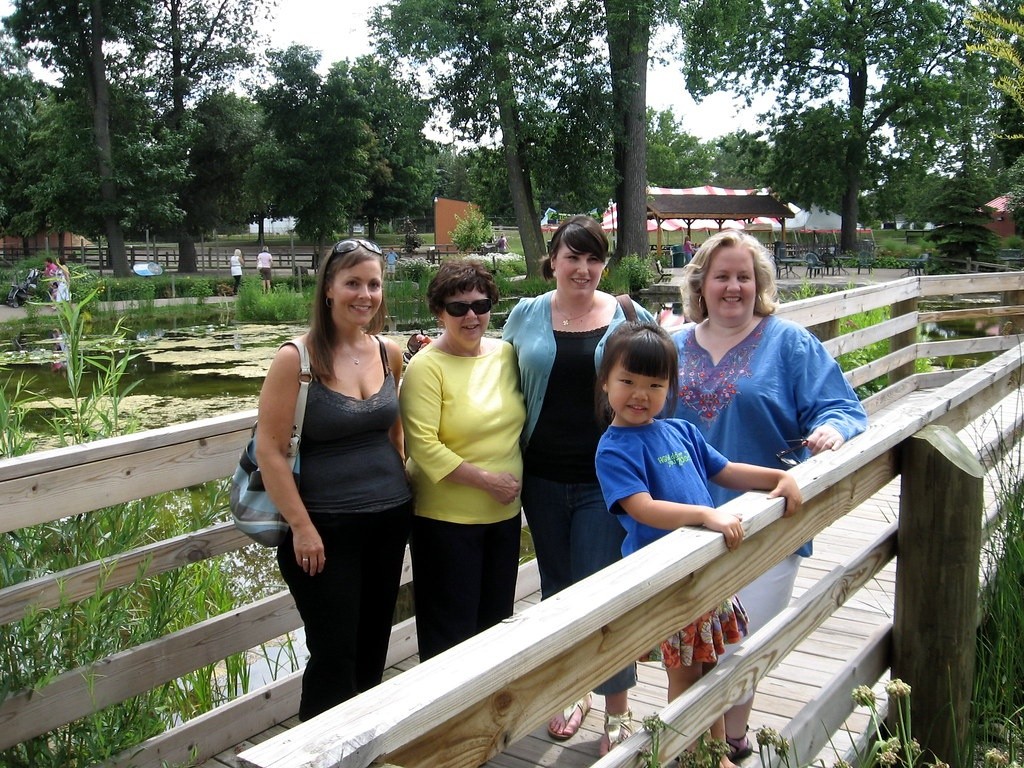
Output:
[340,337,362,364]
[555,293,594,326]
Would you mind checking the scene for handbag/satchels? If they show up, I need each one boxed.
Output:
[229,337,313,547]
[256,260,263,270]
[496,239,501,247]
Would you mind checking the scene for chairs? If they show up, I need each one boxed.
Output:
[773,251,928,280]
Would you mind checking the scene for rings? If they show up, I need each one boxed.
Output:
[828,438,835,446]
[511,498,515,502]
[303,559,308,562]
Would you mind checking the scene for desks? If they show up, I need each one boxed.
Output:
[899,259,925,277]
[779,259,806,278]
[826,256,853,275]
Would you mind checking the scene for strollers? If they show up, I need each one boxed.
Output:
[5,269,42,308]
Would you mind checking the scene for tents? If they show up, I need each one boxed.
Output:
[598,185,876,246]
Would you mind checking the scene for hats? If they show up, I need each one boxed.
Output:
[500,233,506,237]
[60,265,70,274]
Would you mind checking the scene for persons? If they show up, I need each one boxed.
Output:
[669,229,868,760]
[43,257,72,311]
[230,249,244,295]
[496,235,510,252]
[684,234,703,264]
[256,239,413,723]
[398,260,528,663]
[594,323,748,768]
[256,246,273,295]
[503,215,659,757]
[385,247,399,281]
[51,329,67,378]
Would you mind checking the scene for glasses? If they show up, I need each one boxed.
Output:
[403,328,424,365]
[775,442,807,468]
[441,298,493,317]
[323,238,382,286]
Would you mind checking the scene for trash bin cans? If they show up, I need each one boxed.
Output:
[672,245,684,268]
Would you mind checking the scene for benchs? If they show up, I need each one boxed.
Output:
[656,260,674,283]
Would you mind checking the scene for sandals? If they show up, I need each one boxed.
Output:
[547,691,593,740]
[676,733,754,765]
[598,706,633,760]
